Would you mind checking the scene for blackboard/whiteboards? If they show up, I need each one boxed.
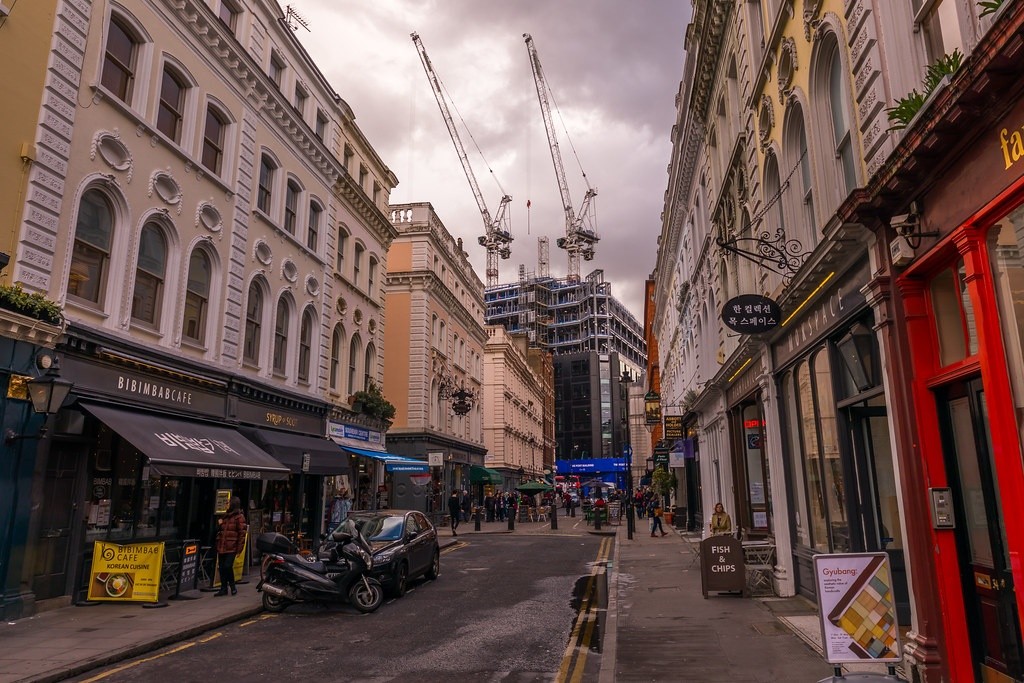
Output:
[518,505,529,519]
[607,502,621,522]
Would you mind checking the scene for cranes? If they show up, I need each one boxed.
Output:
[522,32,602,282]
[410,30,515,286]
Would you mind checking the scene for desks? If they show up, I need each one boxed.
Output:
[743,544,777,588]
[177,547,211,586]
[742,540,770,561]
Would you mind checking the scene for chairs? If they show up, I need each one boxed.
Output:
[746,545,776,598]
[158,546,214,591]
[679,531,701,567]
[528,507,548,522]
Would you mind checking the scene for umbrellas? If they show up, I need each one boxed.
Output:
[580,479,612,493]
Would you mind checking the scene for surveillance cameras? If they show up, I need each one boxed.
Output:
[889,214,916,228]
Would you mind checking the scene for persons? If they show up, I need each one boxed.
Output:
[448,490,462,536]
[213,496,246,597]
[484,489,547,523]
[649,493,668,537]
[594,486,654,520]
[711,503,731,536]
[564,491,572,516]
[459,490,471,524]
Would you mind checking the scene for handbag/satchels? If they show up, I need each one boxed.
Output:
[654,508,663,518]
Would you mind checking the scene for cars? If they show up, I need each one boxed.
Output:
[563,493,580,507]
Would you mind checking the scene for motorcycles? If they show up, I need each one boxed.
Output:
[256,520,383,613]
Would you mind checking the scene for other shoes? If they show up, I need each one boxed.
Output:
[651,533,657,537]
[229,580,237,595]
[452,529,457,536]
[213,590,229,596]
[661,531,668,537]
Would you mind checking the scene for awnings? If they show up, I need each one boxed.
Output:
[253,430,351,475]
[470,466,502,485]
[341,446,429,472]
[80,402,291,481]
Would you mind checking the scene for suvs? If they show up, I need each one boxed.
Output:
[305,509,440,598]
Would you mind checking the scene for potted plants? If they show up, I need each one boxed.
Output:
[0,279,64,350]
[884,47,964,137]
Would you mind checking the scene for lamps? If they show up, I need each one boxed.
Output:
[890,214,939,239]
[5,353,75,446]
[834,323,873,392]
[37,354,52,370]
